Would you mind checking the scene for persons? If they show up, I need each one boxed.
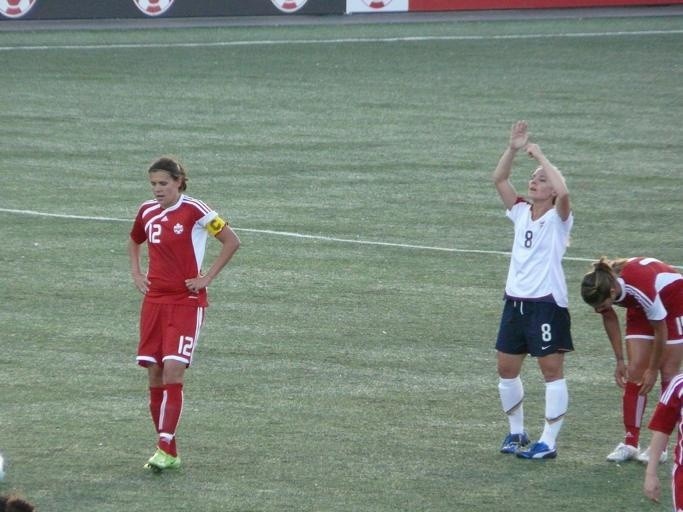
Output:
[128,159,242,473]
[642,374,683,512]
[490,119,576,461]
[579,255,683,465]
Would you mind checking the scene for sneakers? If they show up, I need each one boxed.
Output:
[143,454,181,472]
[147,446,169,474]
[516,440,557,460]
[637,445,668,464]
[606,442,641,462]
[500,431,530,454]
